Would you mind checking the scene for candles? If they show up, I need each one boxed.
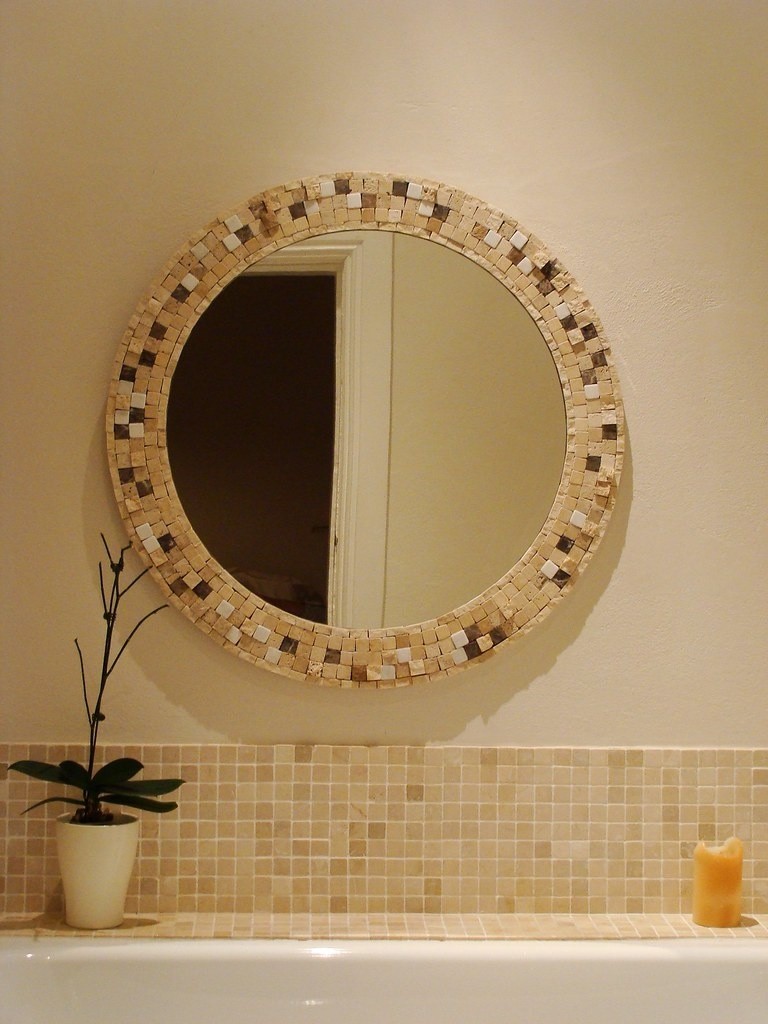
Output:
[694,836,746,929]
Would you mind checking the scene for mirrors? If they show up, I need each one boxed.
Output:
[102,173,625,690]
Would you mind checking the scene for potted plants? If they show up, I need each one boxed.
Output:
[5,531,188,930]
